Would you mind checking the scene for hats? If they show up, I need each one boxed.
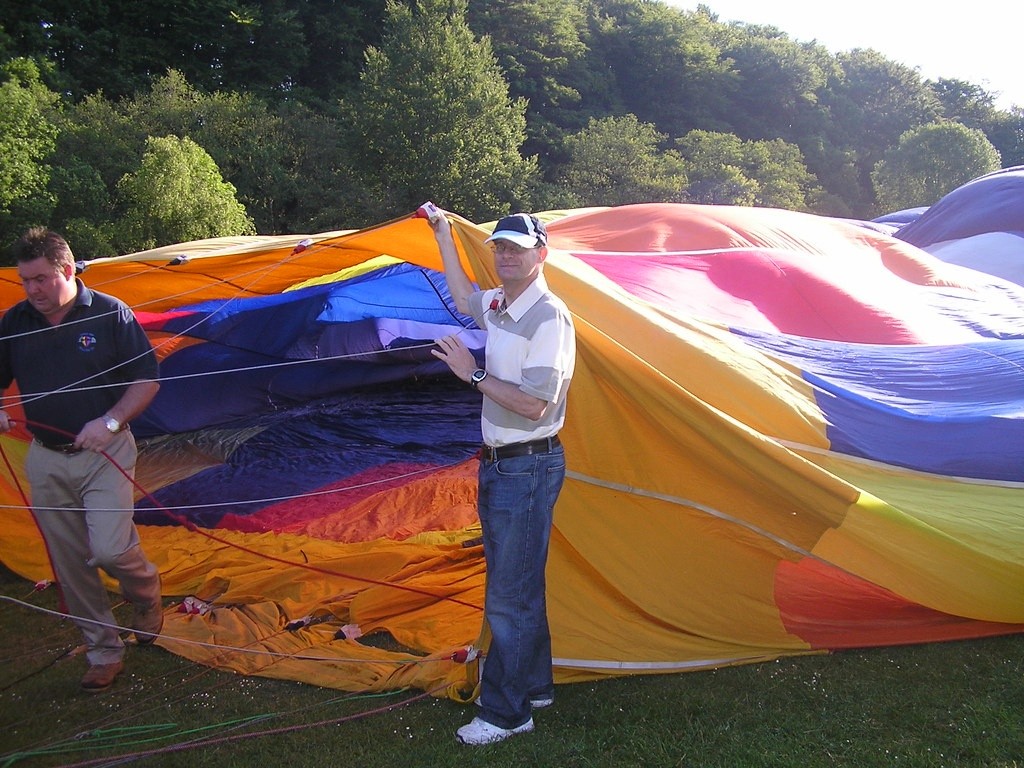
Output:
[484,212,548,248]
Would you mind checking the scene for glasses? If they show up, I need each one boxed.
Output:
[489,242,528,255]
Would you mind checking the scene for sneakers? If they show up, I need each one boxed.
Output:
[79,661,125,693]
[452,716,535,746]
[129,569,164,647]
[474,689,554,708]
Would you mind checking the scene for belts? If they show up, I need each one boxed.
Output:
[479,434,561,462]
[31,433,83,453]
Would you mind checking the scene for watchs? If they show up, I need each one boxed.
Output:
[470,369,488,389]
[101,414,120,434]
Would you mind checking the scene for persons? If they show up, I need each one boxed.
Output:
[428,207,575,746]
[0,228,164,688]
